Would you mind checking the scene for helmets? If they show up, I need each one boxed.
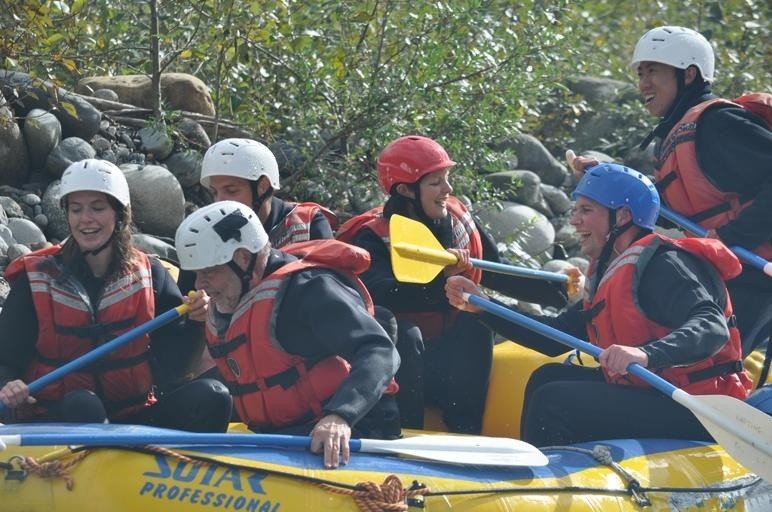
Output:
[201,137,281,193]
[572,164,661,233]
[633,25,715,83]
[58,158,130,213]
[379,135,458,194]
[176,199,268,271]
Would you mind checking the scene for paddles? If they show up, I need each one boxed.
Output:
[461,288,772,487]
[387,213,574,285]
[0,434,549,469]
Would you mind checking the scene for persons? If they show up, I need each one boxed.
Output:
[0,159,233,435]
[339,137,582,436]
[198,139,335,252]
[445,163,752,450]
[173,199,400,471]
[629,25,772,345]
